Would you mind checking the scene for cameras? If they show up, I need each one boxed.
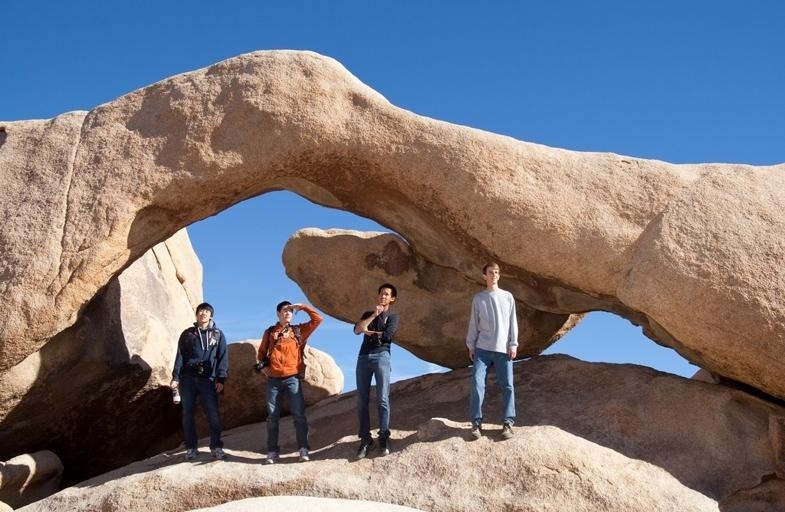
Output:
[369,332,382,347]
[194,361,211,376]
[253,357,268,369]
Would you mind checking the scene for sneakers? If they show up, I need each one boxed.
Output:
[470,422,483,439]
[378,434,391,456]
[185,447,200,461]
[500,421,515,440]
[355,436,376,459]
[209,447,228,460]
[298,447,311,462]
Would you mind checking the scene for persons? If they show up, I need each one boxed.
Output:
[169,301,229,460]
[256,300,325,464]
[465,261,520,440]
[351,284,401,461]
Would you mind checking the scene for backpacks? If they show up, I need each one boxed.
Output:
[267,324,307,379]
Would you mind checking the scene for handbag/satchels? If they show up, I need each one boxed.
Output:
[190,359,214,378]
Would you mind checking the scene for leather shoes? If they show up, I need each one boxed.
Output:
[264,450,281,464]
